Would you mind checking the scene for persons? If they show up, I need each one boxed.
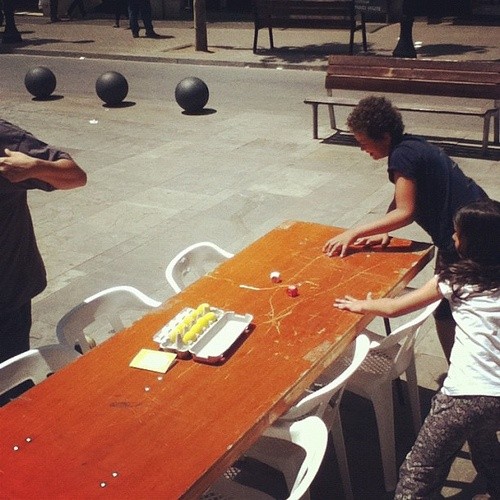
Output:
[112,0,161,39]
[332,198,500,500]
[323,95,499,367]
[65,0,93,20]
[0,118,87,407]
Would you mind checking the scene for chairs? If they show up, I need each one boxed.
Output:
[0,241,442,500]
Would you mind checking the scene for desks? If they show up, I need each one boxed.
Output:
[0,218,434,500]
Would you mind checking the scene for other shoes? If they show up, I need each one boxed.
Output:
[113,24,120,28]
[145,32,161,37]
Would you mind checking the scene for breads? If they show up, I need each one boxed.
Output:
[169,303,217,345]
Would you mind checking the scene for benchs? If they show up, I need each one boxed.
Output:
[252,0,367,54]
[304,55,500,152]
